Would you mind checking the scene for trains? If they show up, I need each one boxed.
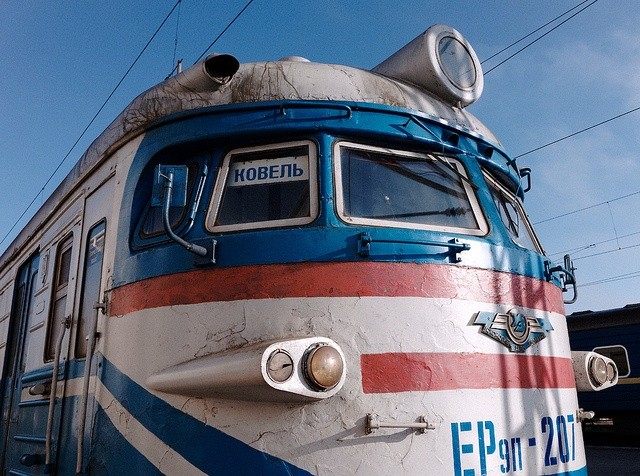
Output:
[564,303,639,436]
[1,23,619,475]
